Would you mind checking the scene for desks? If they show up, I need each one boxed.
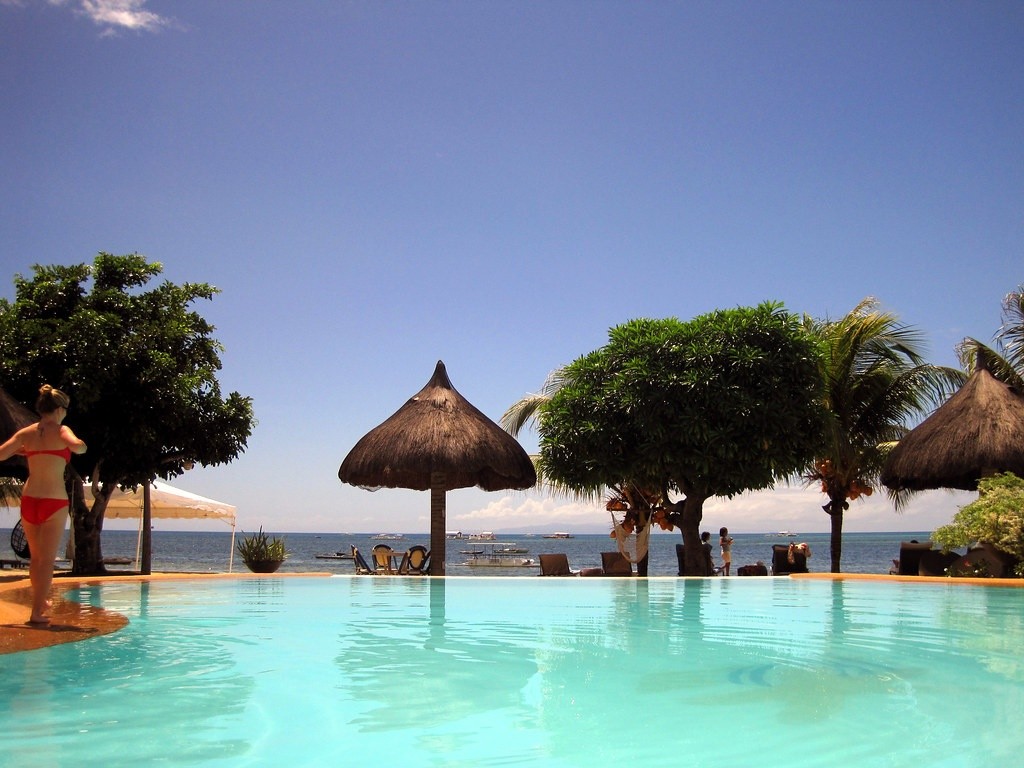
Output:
[373,552,406,573]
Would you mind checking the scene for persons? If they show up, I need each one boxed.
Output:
[717,527,733,576]
[893,540,919,568]
[0,385,87,623]
[700,531,714,575]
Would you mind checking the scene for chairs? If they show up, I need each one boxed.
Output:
[537,554,575,576]
[890,542,932,576]
[772,545,809,575]
[600,552,632,576]
[676,544,724,576]
[350,544,431,576]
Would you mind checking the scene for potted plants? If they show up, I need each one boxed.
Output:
[237,525,293,573]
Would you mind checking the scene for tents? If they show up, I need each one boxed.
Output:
[0,477,24,507]
[83,476,237,572]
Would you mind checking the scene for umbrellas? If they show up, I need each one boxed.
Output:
[880,346,1024,498]
[338,359,537,575]
